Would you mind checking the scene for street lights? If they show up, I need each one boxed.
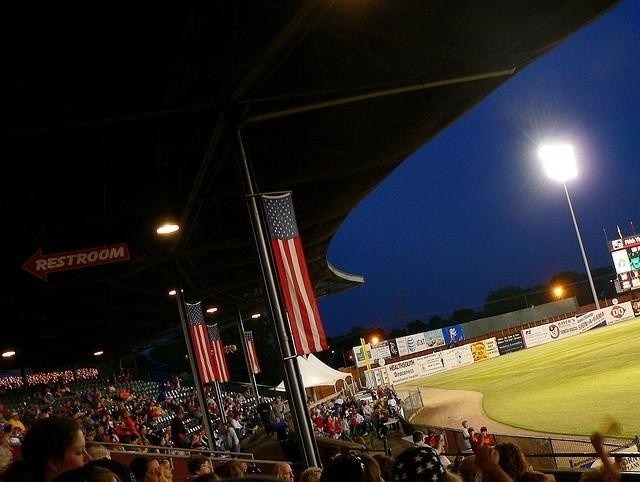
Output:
[533,139,603,313]
[359,335,382,389]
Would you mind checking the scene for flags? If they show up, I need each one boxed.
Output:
[262,193,330,356]
[184,301,231,384]
[243,331,262,375]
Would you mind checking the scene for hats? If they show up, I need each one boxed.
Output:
[393,446,445,481]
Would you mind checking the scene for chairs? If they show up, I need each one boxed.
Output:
[4,380,277,437]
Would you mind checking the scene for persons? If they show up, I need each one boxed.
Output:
[322,421,620,481]
[1,368,321,480]
[311,383,405,446]
